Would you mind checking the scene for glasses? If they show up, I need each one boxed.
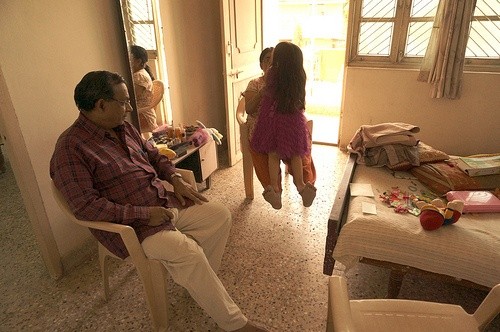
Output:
[109,96,131,108]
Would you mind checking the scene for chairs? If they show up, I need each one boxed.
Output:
[51,168,198,332]
[236,95,313,200]
[326,275,500,332]
[138,80,164,128]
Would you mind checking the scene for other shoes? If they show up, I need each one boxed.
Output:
[231,320,272,332]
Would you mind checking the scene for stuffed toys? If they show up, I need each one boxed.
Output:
[412,199,464,231]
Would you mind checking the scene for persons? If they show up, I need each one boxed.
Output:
[245,41,316,209]
[127,46,160,134]
[49,71,270,332]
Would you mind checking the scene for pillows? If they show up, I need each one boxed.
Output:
[416,199,464,232]
[363,126,500,193]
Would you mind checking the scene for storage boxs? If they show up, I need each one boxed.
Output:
[446,190,500,213]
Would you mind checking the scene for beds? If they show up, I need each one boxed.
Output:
[323,153,499,300]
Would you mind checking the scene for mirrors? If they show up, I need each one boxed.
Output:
[119,1,174,133]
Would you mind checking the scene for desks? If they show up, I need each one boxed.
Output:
[170,135,220,188]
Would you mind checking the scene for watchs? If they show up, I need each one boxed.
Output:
[169,172,182,181]
